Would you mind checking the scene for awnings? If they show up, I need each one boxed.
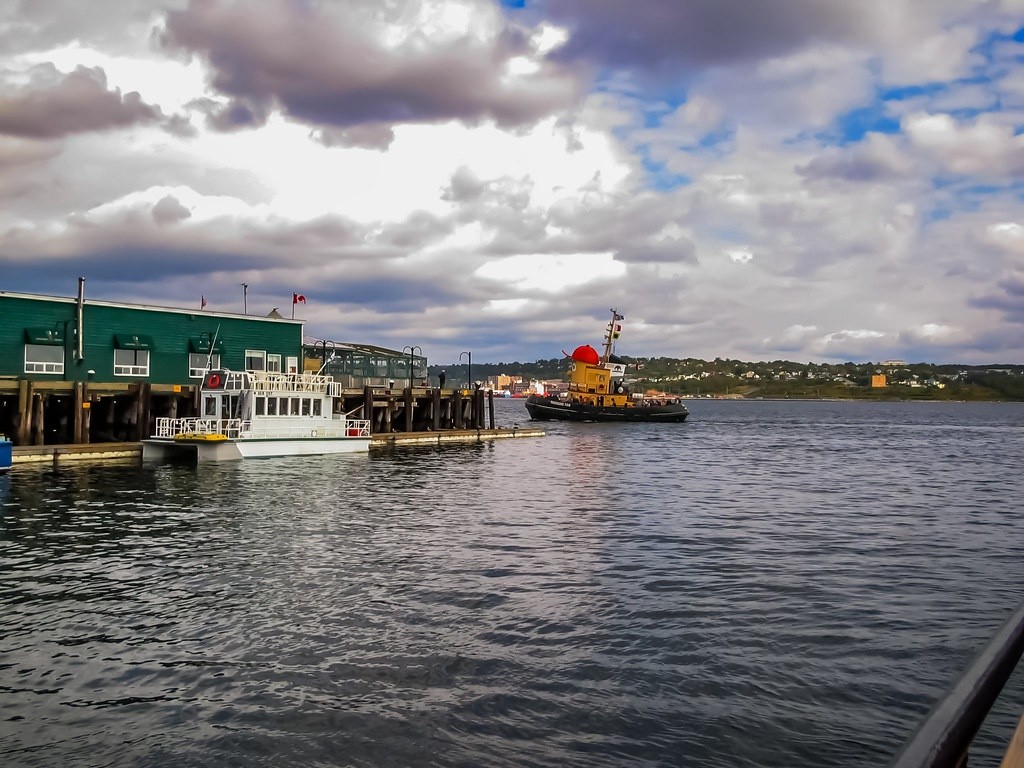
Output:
[188,339,225,354]
[23,327,64,346]
[112,334,155,351]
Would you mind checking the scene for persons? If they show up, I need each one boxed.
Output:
[648,398,661,407]
[438,370,446,388]
[568,394,583,405]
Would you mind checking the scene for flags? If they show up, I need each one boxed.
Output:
[612,332,620,340]
[293,294,307,305]
[614,323,622,332]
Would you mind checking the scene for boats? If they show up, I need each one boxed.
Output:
[523,308,690,422]
[138,367,372,463]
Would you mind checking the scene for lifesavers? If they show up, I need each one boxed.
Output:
[208,375,220,389]
